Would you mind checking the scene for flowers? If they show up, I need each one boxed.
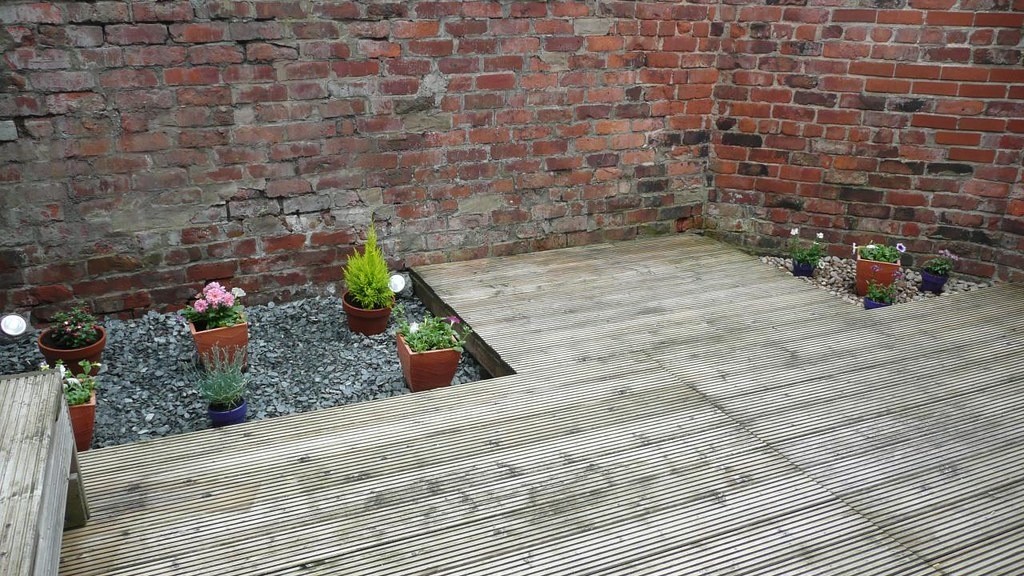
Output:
[788,227,825,267]
[863,263,904,304]
[37,358,108,406]
[921,249,960,276]
[851,239,907,263]
[179,281,248,330]
[391,301,474,354]
[46,300,105,348]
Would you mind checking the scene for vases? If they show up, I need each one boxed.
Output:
[856,253,900,297]
[793,257,817,277]
[920,268,950,294]
[37,322,105,378]
[396,330,464,392]
[190,313,247,370]
[864,297,892,310]
[68,390,95,452]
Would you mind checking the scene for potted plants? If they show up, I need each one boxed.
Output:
[192,339,248,428]
[343,220,395,337]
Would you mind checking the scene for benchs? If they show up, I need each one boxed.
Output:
[0,369,90,576]
